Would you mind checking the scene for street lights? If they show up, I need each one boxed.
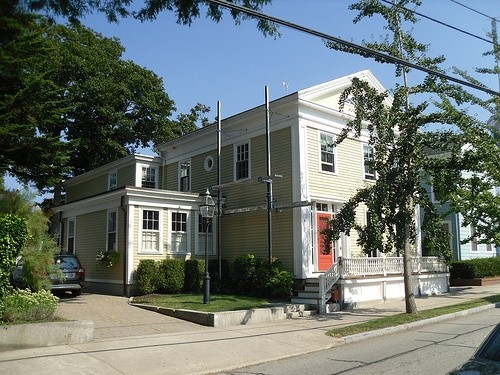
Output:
[197,188,215,304]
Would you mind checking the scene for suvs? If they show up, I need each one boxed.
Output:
[10,250,86,296]
